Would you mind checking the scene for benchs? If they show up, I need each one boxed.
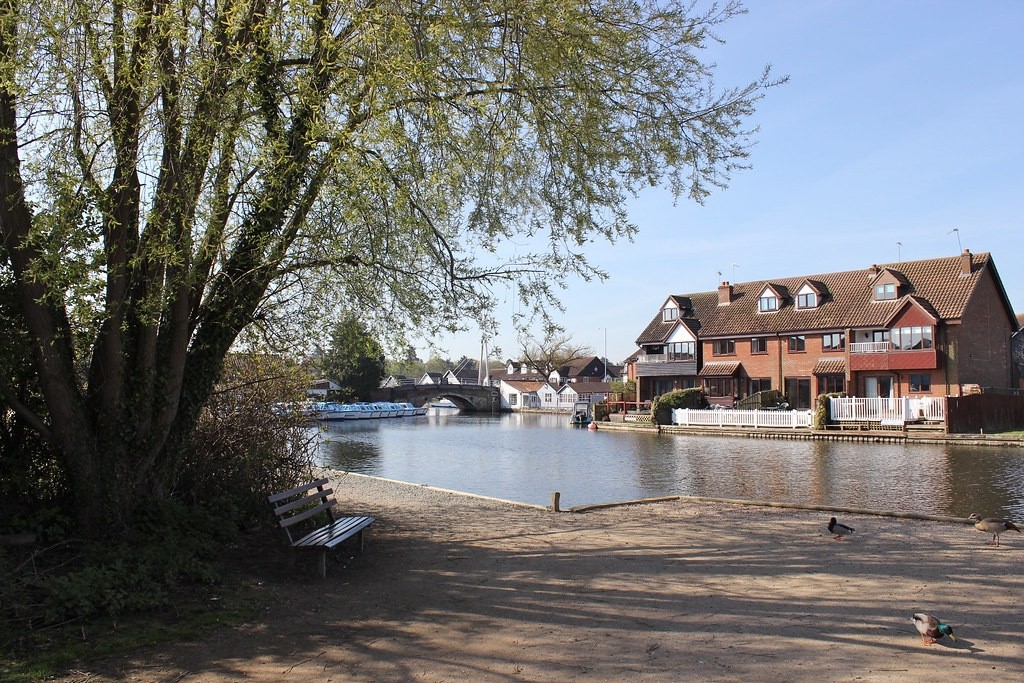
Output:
[265,478,375,576]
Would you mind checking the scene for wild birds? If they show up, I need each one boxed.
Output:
[827,517,855,540]
[910,611,958,647]
[967,512,1023,548]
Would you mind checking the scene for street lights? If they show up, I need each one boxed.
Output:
[596,327,607,382]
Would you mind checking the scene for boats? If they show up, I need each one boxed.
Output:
[271,402,429,422]
[570,401,592,425]
[431,398,458,408]
